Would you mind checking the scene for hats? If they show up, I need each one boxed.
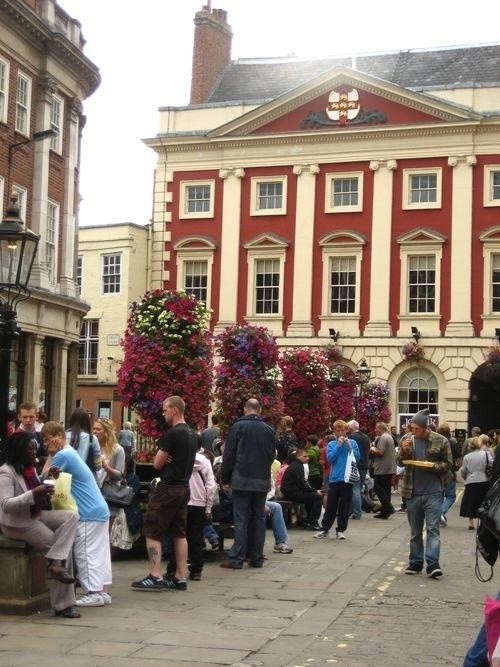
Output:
[409,407,430,426]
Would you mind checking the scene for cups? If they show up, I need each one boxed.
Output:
[44,478,57,496]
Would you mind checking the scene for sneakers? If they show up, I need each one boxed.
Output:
[438,512,447,527]
[188,571,202,581]
[273,543,294,555]
[301,519,346,541]
[211,537,219,549]
[403,563,443,579]
[74,591,113,607]
[130,574,189,592]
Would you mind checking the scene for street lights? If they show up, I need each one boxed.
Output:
[1,190,39,434]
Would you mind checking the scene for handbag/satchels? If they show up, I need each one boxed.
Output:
[49,472,78,514]
[483,450,493,478]
[86,434,96,475]
[100,469,135,506]
[475,479,500,540]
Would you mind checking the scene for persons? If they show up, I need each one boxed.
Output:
[1,397,500,667]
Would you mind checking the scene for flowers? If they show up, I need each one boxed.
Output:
[402,341,425,367]
[318,341,343,364]
[484,341,499,369]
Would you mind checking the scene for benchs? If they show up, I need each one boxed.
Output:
[208,521,233,554]
[279,500,305,529]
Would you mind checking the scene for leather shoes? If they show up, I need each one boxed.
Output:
[45,564,75,584]
[54,606,82,619]
[374,506,396,520]
[220,558,263,569]
